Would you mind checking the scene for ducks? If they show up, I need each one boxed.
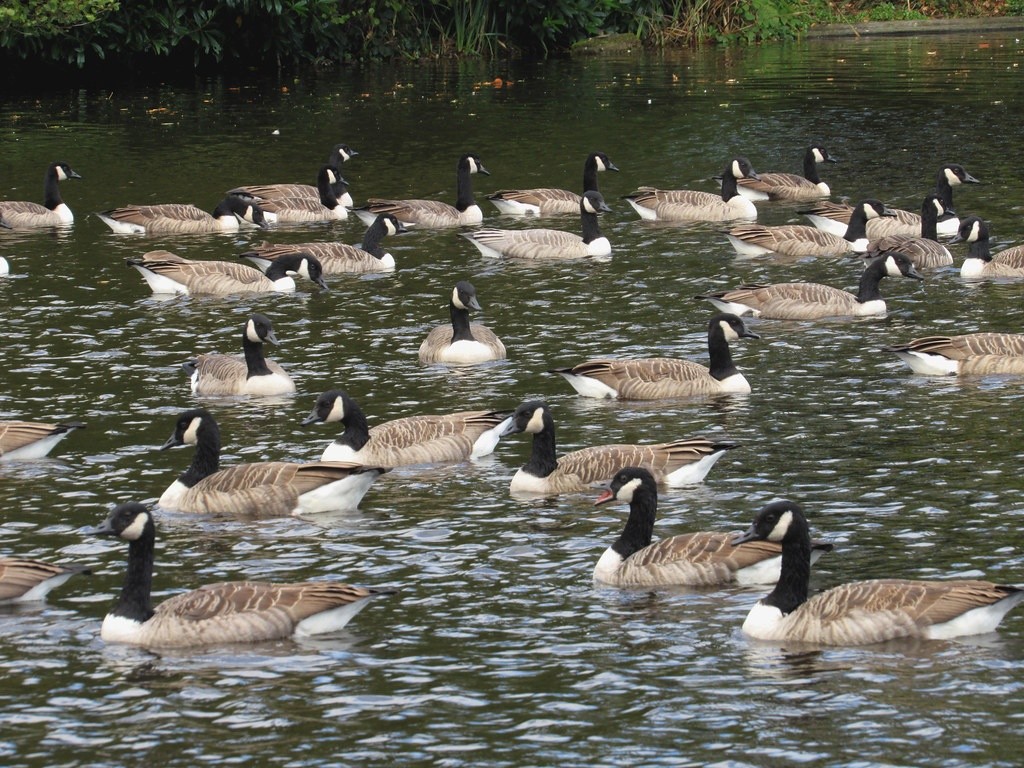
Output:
[155,408,395,518]
[181,312,297,397]
[418,280,507,364]
[1,418,88,460]
[499,400,744,500]
[300,387,518,467]
[594,466,834,588]
[0,554,94,608]
[547,312,761,399]
[729,497,1024,644]
[1,142,1023,326]
[881,332,1024,377]
[75,500,398,649]
